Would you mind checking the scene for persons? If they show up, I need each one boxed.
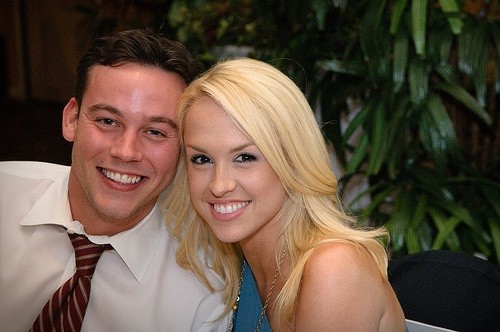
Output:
[0,29,235,332]
[159,56,407,332]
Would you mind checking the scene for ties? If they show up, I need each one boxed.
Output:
[28,228,113,332]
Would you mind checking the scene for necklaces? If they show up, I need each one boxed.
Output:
[225,242,286,332]
